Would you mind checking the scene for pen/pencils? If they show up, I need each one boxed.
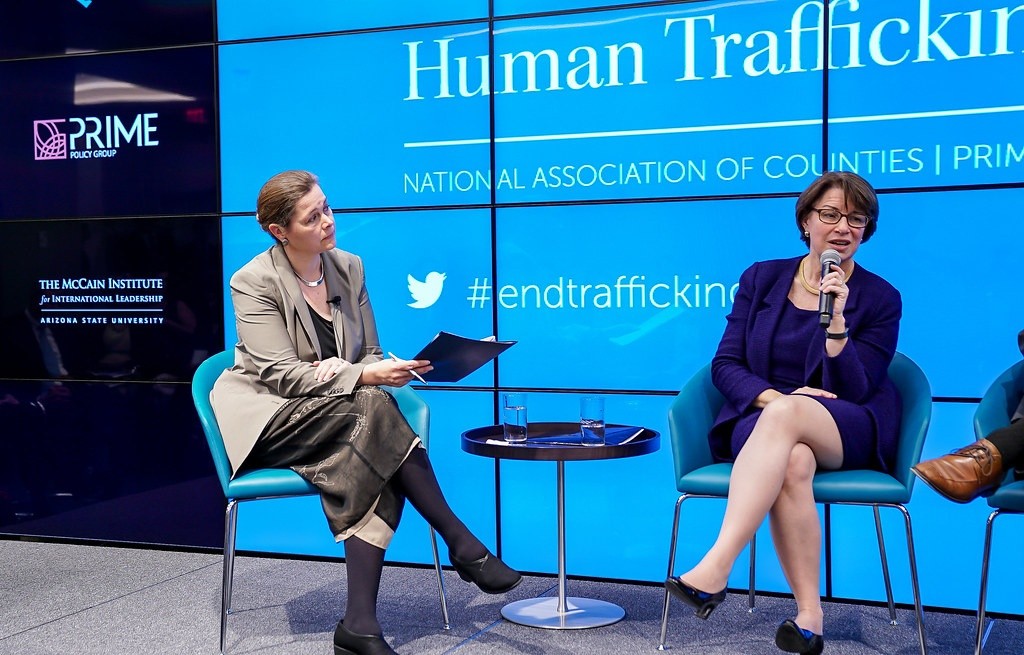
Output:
[388,351,428,386]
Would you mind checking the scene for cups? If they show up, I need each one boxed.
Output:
[580,396,606,447]
[501,392,529,442]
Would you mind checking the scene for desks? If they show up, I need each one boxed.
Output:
[461,422,660,630]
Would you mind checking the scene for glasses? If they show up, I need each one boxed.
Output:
[812,209,869,229]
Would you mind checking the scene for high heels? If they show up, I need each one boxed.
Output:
[666,576,727,619]
[775,620,824,655]
[449,550,524,594]
[332,620,397,655]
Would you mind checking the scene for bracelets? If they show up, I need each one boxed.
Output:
[825,327,849,339]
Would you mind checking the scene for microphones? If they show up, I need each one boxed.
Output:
[818,248,841,328]
[333,295,341,302]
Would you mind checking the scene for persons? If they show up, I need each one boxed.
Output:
[910,329,1024,504]
[665,171,903,655]
[208,171,525,655]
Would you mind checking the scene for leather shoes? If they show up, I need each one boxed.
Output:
[910,439,1002,503]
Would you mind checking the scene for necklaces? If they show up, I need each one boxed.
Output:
[799,261,851,295]
[293,258,325,286]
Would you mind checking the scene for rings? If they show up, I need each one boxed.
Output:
[838,280,844,287]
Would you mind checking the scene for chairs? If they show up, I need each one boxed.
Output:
[972,358,1024,655]
[191,348,449,655]
[657,349,932,655]
